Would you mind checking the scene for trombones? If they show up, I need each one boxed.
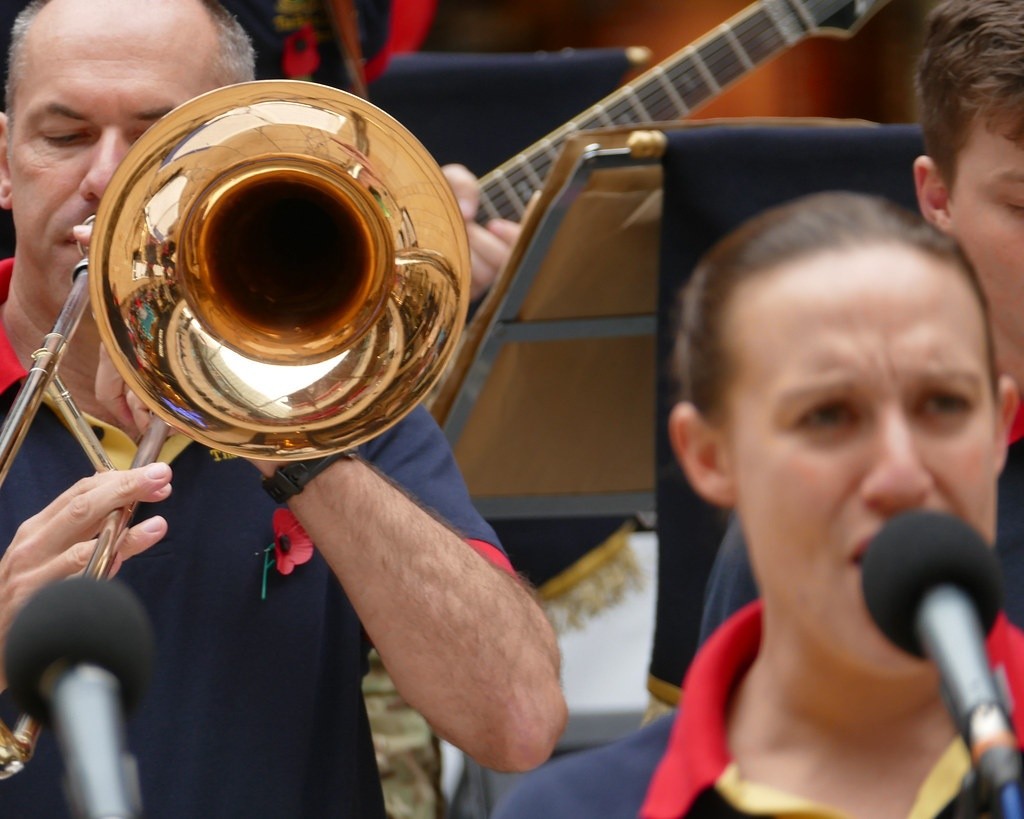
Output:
[1,77,475,781]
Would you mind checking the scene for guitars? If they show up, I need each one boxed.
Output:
[463,2,892,243]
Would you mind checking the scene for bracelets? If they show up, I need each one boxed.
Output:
[261,454,354,504]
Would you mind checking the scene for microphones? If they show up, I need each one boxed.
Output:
[5,575,154,819]
[862,509,1024,819]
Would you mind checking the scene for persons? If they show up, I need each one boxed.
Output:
[0,0,571,819]
[690,1,1024,663]
[490,187,1024,819]
[211,0,577,304]
